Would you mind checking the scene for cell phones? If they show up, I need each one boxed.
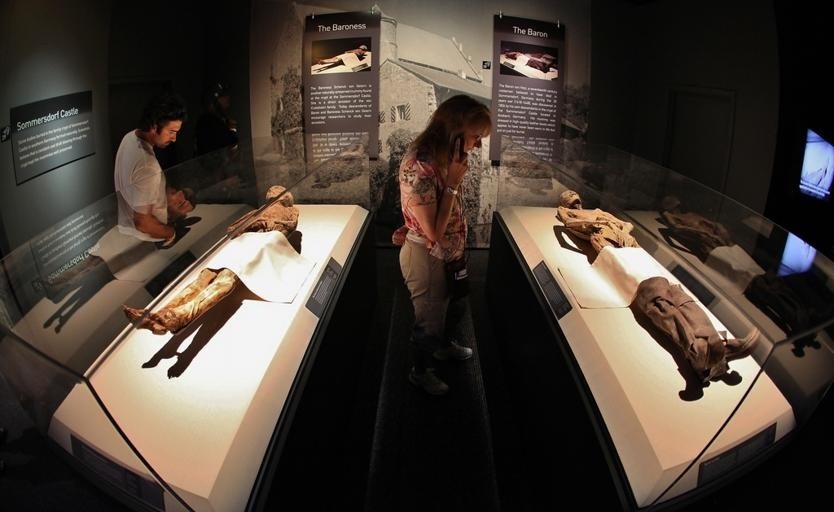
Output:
[451,131,465,159]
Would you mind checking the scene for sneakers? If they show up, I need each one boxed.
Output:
[432,340,473,362]
[693,334,725,385]
[408,368,449,395]
[723,328,760,363]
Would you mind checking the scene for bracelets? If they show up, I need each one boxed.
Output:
[443,185,458,195]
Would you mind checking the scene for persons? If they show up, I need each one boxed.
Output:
[555,190,762,384]
[659,195,767,295]
[391,95,491,395]
[120,184,299,335]
[166,186,194,220]
[318,45,368,64]
[113,91,188,248]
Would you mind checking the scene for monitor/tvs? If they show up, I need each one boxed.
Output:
[794,124,834,208]
[774,232,817,281]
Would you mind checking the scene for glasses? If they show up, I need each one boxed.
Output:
[472,133,482,141]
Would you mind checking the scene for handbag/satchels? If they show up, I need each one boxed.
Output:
[445,256,470,297]
